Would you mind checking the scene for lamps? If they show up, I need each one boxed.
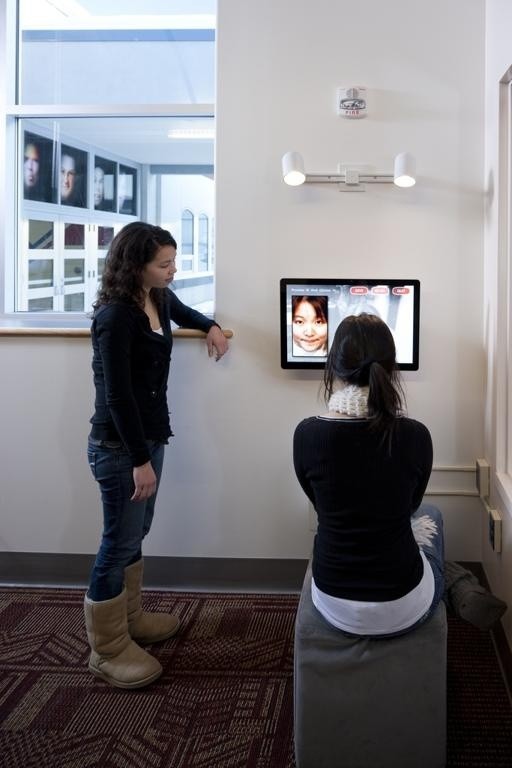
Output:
[281,151,416,192]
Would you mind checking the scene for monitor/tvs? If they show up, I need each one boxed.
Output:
[279,276,421,371]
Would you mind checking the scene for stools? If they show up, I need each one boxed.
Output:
[294,561,447,766]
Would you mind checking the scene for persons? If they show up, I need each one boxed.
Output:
[23,143,41,190]
[81,219,232,695]
[290,310,446,643]
[59,152,76,200]
[290,293,330,359]
[93,165,104,207]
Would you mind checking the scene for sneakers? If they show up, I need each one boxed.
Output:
[453,586,510,634]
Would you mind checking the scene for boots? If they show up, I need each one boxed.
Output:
[124,555,184,645]
[78,580,164,691]
